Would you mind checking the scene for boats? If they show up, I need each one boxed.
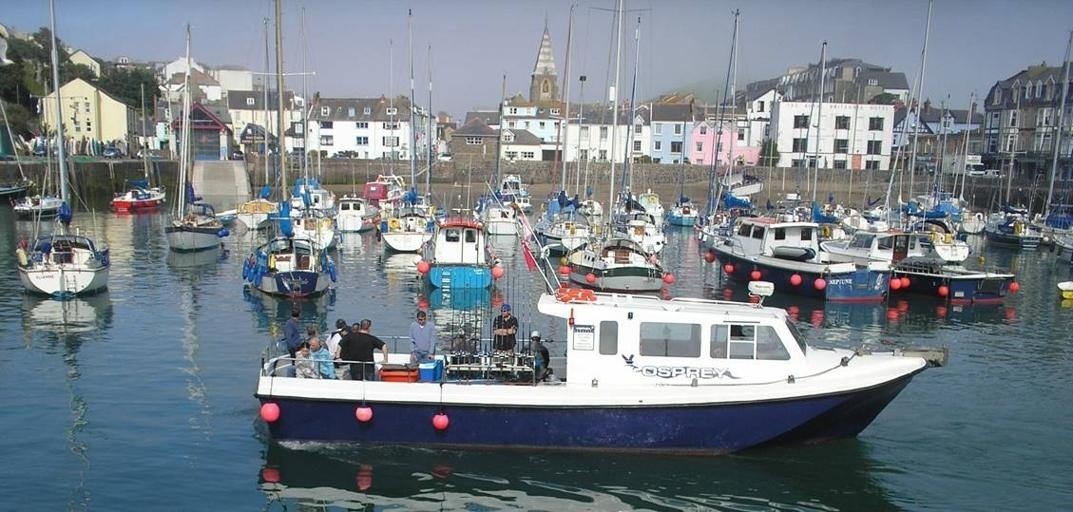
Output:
[253,290,956,456]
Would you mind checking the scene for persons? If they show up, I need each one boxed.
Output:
[492,304,519,381]
[494,189,502,202]
[282,307,388,381]
[480,195,486,208]
[408,312,437,367]
[509,201,517,219]
[519,331,549,382]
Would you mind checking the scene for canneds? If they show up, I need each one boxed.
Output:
[1014,224,1021,234]
[822,226,829,237]
[649,196,656,203]
[389,218,400,229]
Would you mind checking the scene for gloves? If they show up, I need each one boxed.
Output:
[253,290,956,456]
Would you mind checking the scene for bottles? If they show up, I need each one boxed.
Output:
[531,330,541,338]
[501,304,511,311]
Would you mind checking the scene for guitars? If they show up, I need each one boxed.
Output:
[432,413,448,432]
[435,464,450,480]
[720,289,1016,326]
[586,272,595,283]
[491,265,504,277]
[357,472,374,491]
[417,297,429,308]
[262,467,281,483]
[261,402,280,423]
[418,261,430,275]
[559,265,569,273]
[491,296,504,307]
[664,274,674,286]
[356,406,372,422]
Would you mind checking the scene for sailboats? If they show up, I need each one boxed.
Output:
[13,76,63,220]
[110,82,166,215]
[163,22,228,252]
[2,104,34,199]
[15,2,112,299]
[237,0,1073,313]
